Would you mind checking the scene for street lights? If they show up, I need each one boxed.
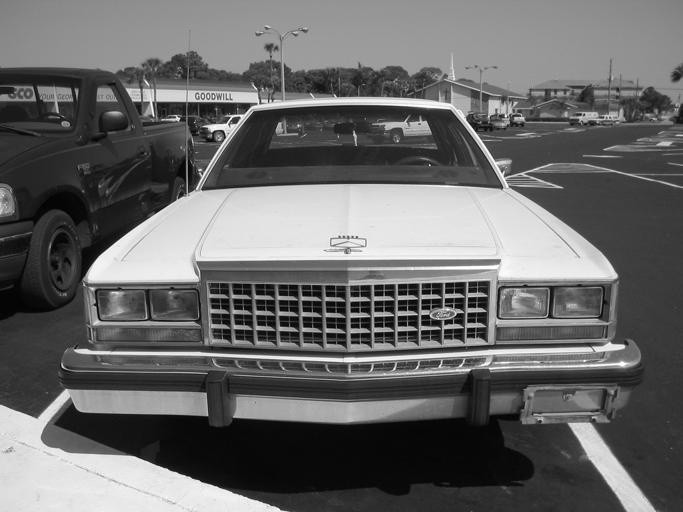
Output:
[255,24,309,101]
[465,64,498,112]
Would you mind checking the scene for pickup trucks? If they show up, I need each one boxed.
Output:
[0,66,198,311]
[367,110,432,145]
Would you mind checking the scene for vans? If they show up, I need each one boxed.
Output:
[568,111,599,126]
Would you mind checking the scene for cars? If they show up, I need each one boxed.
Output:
[465,112,525,132]
[160,113,305,142]
[59,96,644,424]
[595,115,620,127]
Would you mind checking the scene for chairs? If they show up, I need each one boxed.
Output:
[269,146,442,166]
